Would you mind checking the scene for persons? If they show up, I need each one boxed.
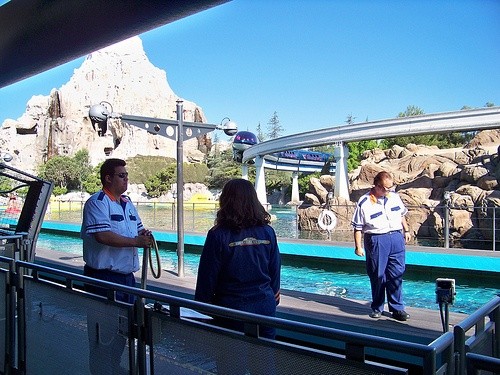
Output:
[350,171,411,321]
[79,158,153,375]
[193,178,282,375]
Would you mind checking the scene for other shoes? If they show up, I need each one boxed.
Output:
[393,310,410,319]
[370,308,382,317]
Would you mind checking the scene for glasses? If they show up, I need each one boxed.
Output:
[379,184,395,191]
[114,172,129,178]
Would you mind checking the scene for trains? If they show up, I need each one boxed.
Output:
[231,132,339,177]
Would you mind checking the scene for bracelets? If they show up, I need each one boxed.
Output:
[405,230,409,232]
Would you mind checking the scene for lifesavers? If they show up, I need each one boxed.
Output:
[318,211,336,230]
